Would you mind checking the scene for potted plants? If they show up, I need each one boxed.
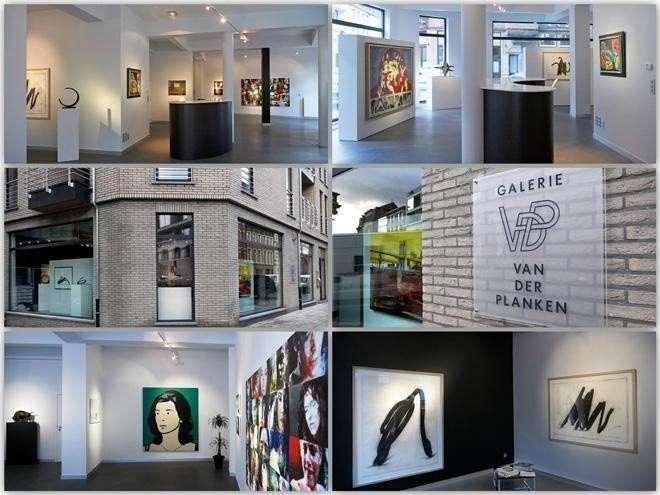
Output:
[207,413,230,471]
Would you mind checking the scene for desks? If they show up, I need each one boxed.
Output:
[6,422,40,466]
[493,464,536,491]
[168,100,233,160]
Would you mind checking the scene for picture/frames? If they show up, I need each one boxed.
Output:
[127,68,141,98]
[214,81,223,95]
[598,30,625,77]
[54,266,73,290]
[26,67,51,119]
[542,52,571,81]
[168,79,186,95]
[352,364,446,489]
[365,41,415,121]
[547,368,638,455]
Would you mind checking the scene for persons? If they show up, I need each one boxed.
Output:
[142,389,198,452]
[234,331,328,494]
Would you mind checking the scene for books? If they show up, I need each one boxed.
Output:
[496,462,535,479]
